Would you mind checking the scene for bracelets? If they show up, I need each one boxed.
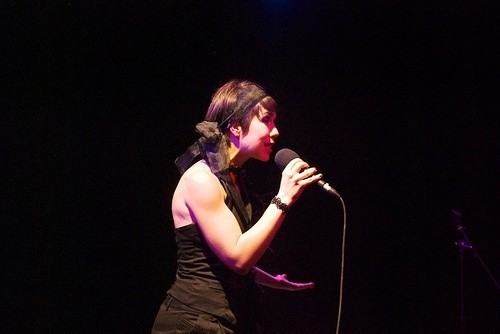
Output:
[271,196,290,215]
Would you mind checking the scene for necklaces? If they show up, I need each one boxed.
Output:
[227,163,244,177]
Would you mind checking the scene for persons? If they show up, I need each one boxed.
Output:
[149,76,323,334]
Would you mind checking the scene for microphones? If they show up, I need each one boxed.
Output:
[275,149,331,191]
